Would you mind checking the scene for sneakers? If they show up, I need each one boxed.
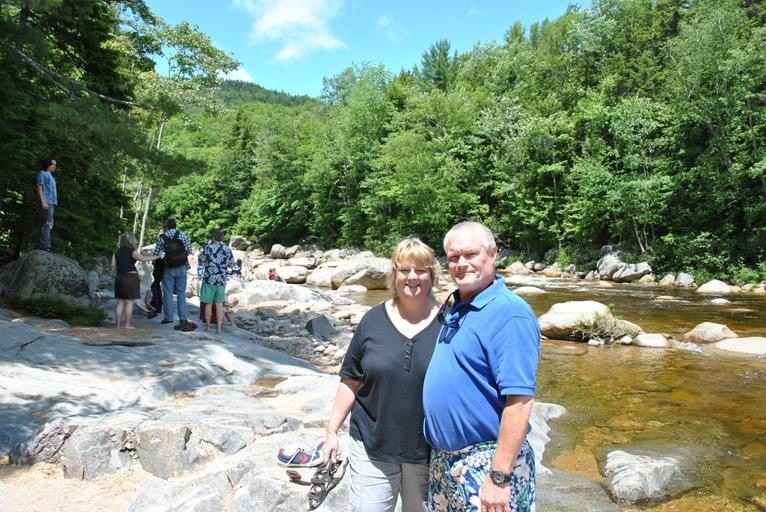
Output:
[161,318,174,324]
[278,442,331,468]
[175,319,188,330]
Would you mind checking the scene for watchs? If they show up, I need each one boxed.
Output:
[485,467,515,484]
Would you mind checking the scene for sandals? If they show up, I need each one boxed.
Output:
[308,459,349,510]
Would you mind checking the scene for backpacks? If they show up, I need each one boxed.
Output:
[161,230,187,267]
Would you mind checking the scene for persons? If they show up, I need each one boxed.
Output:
[36,157,59,255]
[268,268,282,282]
[421,218,541,511]
[199,299,240,330]
[197,229,235,335]
[153,219,194,325]
[135,283,157,314]
[327,236,445,511]
[110,232,164,330]
[232,259,242,275]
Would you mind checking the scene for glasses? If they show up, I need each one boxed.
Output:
[438,291,466,329]
